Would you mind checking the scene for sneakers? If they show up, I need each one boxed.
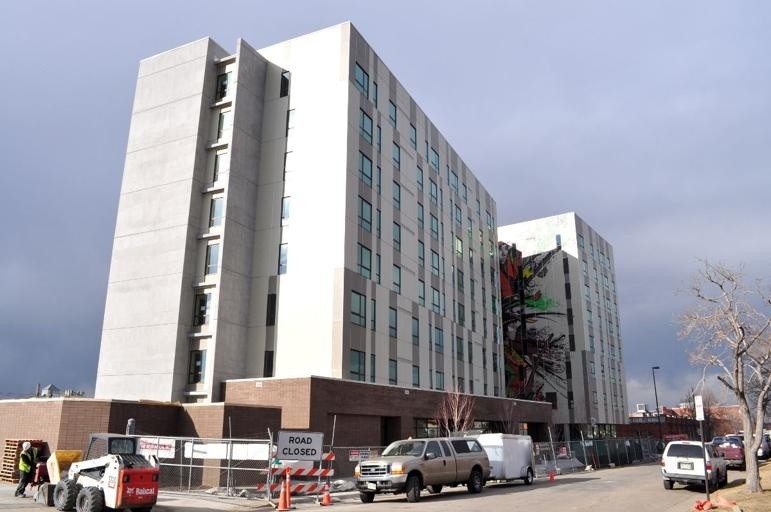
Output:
[15,493,26,498]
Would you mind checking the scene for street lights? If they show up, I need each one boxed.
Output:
[653,366,664,437]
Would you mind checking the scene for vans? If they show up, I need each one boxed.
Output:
[661,439,728,492]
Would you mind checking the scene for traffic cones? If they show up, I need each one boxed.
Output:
[549,467,556,481]
[276,480,291,510]
[320,482,335,505]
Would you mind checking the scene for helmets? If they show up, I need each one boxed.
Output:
[22,442,31,451]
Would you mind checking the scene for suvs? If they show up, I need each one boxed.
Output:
[353,437,495,502]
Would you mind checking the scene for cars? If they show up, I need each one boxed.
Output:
[712,428,771,467]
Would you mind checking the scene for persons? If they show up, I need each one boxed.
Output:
[12,440,39,499]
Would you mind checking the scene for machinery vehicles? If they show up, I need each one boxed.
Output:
[32,433,162,511]
